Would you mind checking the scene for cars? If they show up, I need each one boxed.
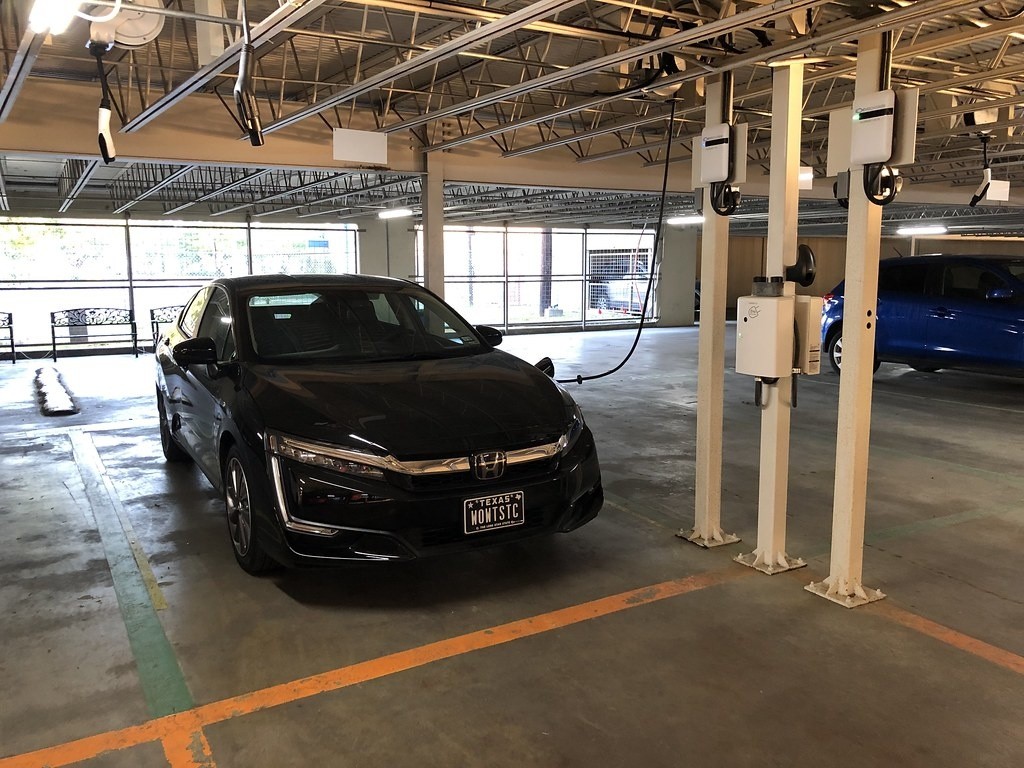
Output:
[819,254,1024,379]
[155,274,605,579]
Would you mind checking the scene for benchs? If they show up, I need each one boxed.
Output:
[150,305,187,353]
[0,312,16,364]
[50,308,138,362]
